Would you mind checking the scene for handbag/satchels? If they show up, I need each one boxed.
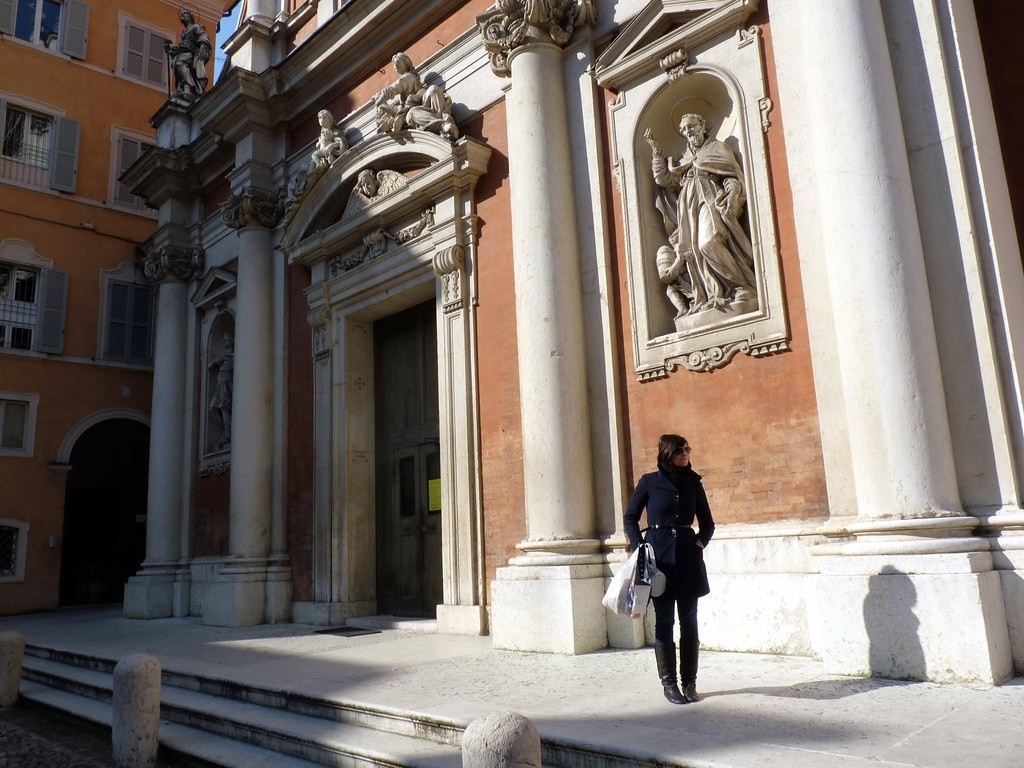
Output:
[602,544,652,619]
[641,543,666,597]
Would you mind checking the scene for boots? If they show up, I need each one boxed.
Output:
[680,639,700,702]
[654,642,686,704]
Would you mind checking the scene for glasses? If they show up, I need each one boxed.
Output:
[675,447,692,455]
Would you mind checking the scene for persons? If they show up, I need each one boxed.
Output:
[358,170,377,196]
[164,9,213,96]
[643,112,741,319]
[312,109,338,168]
[376,53,459,137]
[623,435,716,705]
[208,332,234,447]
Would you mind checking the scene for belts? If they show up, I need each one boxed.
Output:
[640,525,691,533]
